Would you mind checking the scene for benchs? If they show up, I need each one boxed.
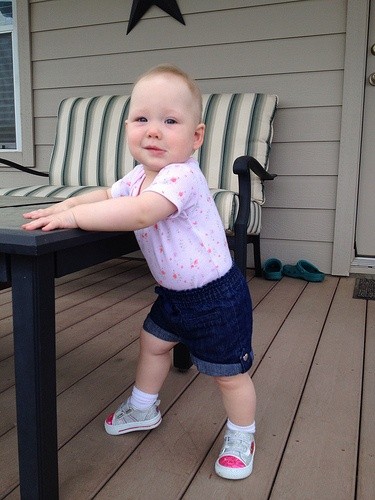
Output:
[0,92,279,282]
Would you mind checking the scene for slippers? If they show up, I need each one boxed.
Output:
[283,259,325,281]
[262,258,283,280]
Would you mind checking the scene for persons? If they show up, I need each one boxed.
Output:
[22,64,257,480]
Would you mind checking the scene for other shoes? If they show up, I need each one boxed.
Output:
[214,430,256,479]
[104,396,162,435]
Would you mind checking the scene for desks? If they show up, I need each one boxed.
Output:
[0,195,193,500]
